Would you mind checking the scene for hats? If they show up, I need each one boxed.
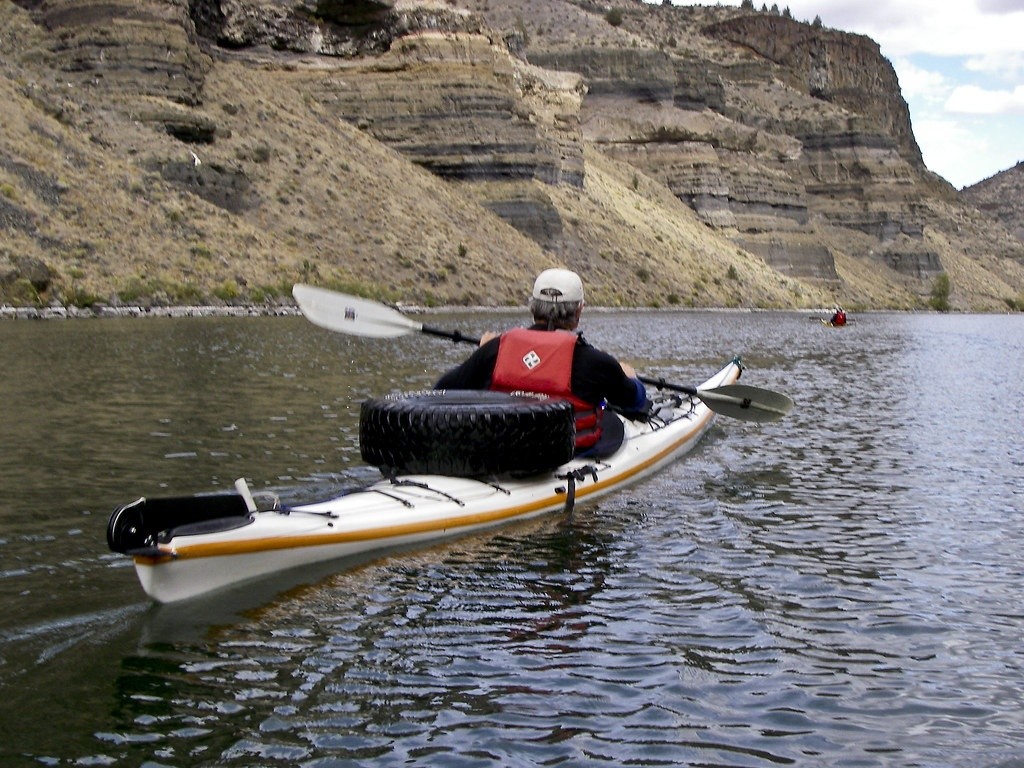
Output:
[533,269,584,302]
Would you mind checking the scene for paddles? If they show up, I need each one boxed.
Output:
[292,283,794,422]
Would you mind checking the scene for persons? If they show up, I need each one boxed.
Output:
[831,307,846,323]
[435,268,646,457]
